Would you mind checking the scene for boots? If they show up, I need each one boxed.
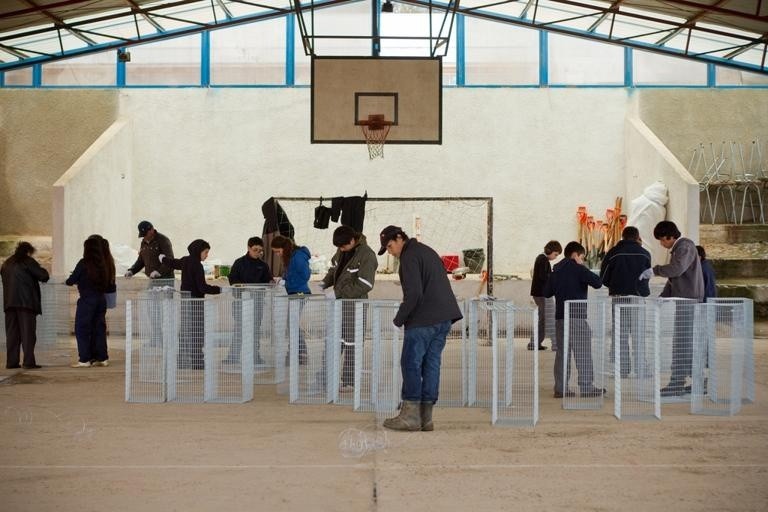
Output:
[421,400,434,431]
[383,400,422,431]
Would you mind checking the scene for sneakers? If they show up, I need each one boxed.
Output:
[527,344,547,350]
[6,365,21,369]
[23,365,42,369]
[340,384,355,393]
[553,390,577,398]
[91,360,109,366]
[660,387,685,396]
[581,388,606,396]
[686,384,708,394]
[70,361,90,368]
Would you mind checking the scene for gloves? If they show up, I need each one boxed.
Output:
[278,279,285,288]
[639,268,654,280]
[124,271,133,277]
[326,291,336,300]
[159,254,166,263]
[317,282,325,291]
[150,270,161,278]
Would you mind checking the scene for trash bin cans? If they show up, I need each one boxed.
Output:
[214,265,229,278]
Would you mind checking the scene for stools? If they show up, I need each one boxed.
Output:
[688,139,768,227]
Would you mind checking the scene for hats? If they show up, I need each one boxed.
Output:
[378,225,402,255]
[138,221,153,237]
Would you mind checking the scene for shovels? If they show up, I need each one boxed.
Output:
[578,206,627,269]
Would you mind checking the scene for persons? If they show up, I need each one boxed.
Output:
[599,227,658,379]
[271,234,314,368]
[543,241,607,400]
[319,224,379,394]
[155,239,224,372]
[695,246,719,395]
[86,233,117,335]
[0,241,50,369]
[628,233,643,339]
[377,226,465,432]
[65,238,109,368]
[639,220,706,397]
[527,238,562,351]
[124,220,174,349]
[221,236,275,365]
